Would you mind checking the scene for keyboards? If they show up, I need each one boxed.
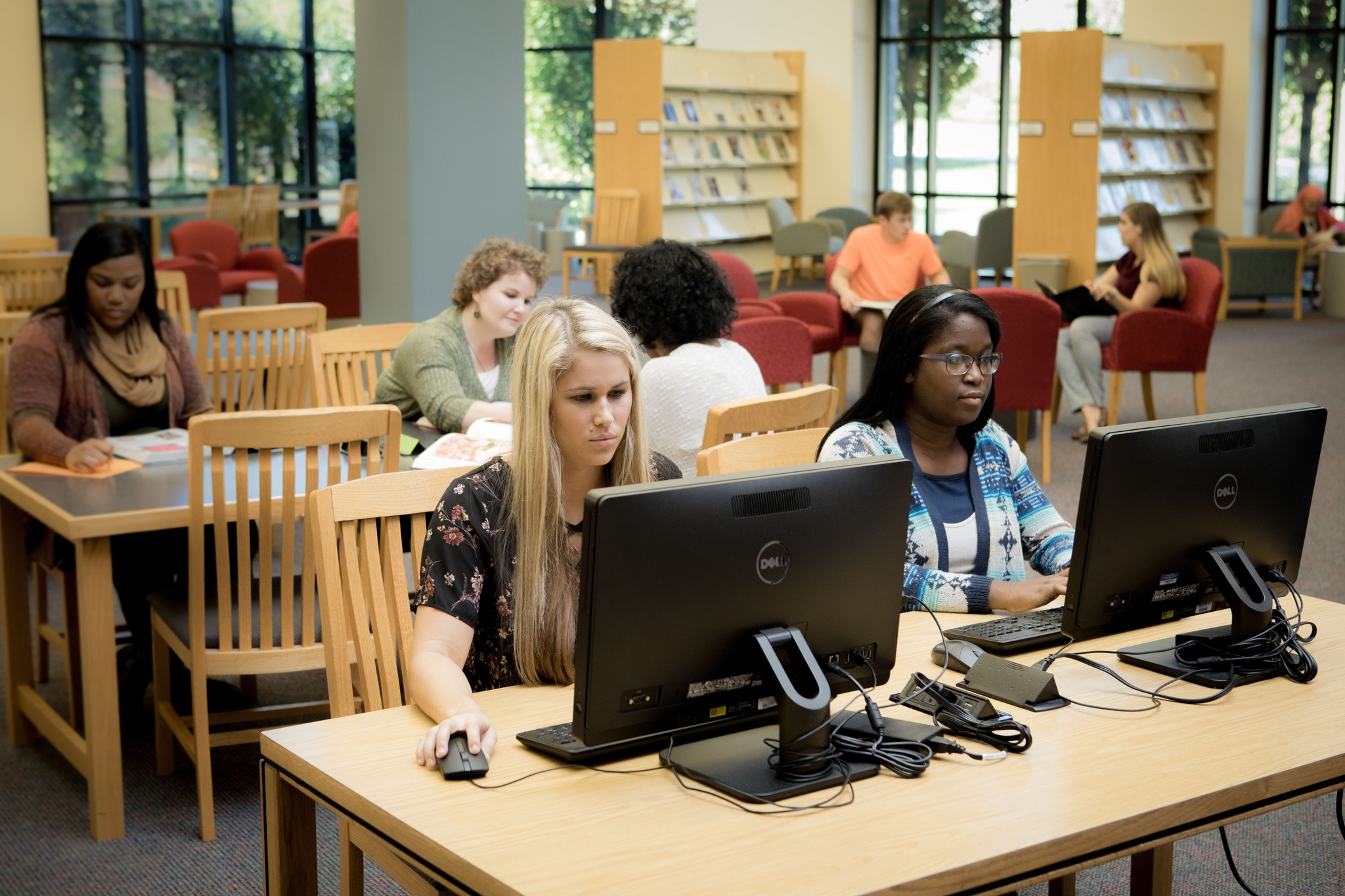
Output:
[943,606,1064,657]
[519,705,780,772]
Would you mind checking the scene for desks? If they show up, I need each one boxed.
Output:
[258,593,1345,896]
[0,425,443,841]
[111,200,340,259]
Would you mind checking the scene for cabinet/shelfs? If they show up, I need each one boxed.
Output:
[1013,31,1226,295]
[592,39,807,296]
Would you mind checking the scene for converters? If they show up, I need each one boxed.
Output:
[826,708,946,755]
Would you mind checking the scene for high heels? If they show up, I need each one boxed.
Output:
[1072,407,1108,439]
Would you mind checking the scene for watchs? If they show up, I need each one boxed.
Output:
[1104,287,1117,301]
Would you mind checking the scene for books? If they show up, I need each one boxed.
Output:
[104,427,234,465]
[411,417,513,470]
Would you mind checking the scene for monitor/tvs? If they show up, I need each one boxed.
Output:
[1061,401,1329,689]
[572,456,914,803]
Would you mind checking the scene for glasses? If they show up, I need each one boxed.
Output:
[920,353,1000,375]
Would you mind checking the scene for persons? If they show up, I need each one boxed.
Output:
[1056,202,1188,443]
[4,220,259,717]
[830,193,952,396]
[608,237,774,479]
[815,285,1075,615]
[409,298,681,770]
[340,239,552,553]
[1273,185,1345,311]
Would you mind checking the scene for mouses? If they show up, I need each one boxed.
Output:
[434,735,492,780]
[929,637,990,674]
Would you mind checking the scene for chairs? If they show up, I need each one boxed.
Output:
[0,178,1345,894]
